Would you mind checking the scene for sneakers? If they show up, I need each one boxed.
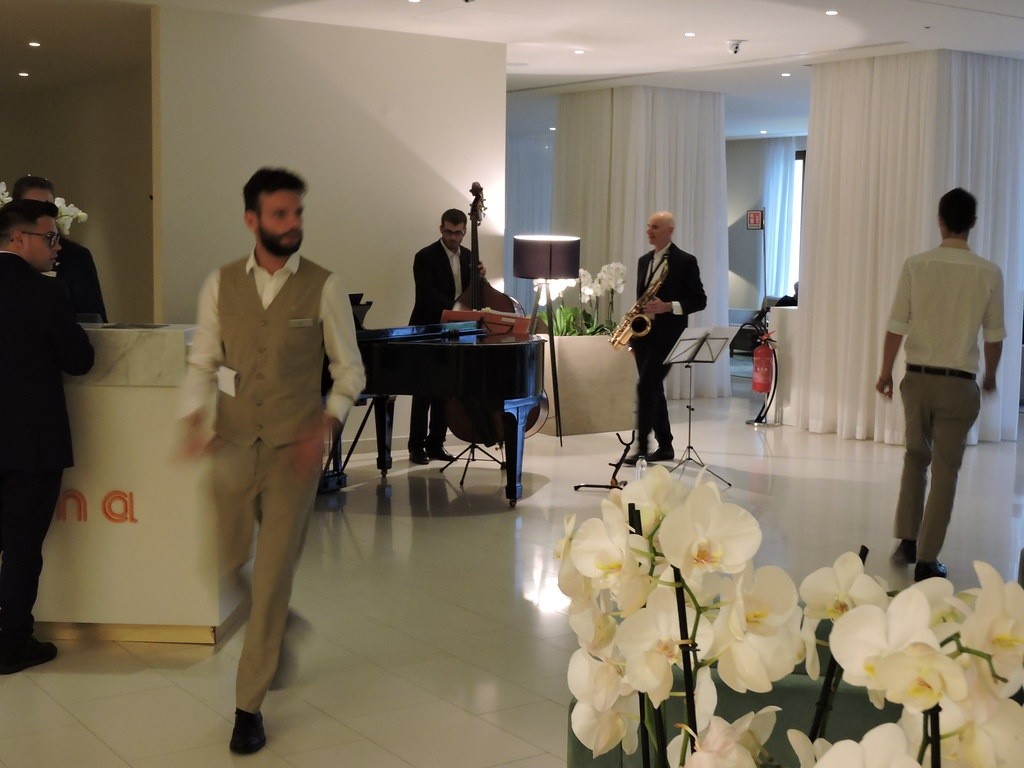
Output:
[228,706,266,755]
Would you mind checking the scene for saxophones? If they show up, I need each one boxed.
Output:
[607,262,669,352]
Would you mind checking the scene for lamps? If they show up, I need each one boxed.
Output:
[513,234,581,447]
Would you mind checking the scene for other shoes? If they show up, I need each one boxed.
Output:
[628,453,648,459]
[0,641,58,675]
[914,561,949,582]
[889,541,917,565]
[648,446,675,461]
[425,446,453,461]
[409,448,430,464]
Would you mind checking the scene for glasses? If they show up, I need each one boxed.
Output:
[442,227,467,235]
[10,231,63,248]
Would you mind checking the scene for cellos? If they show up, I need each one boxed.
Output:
[442,181,549,470]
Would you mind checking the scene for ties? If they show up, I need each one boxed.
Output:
[642,256,665,291]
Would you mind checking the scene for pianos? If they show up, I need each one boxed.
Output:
[321,293,548,508]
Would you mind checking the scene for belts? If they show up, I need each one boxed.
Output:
[906,362,976,381]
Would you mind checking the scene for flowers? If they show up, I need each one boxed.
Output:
[0,174,88,234]
[556,461,1024,768]
[533,259,626,334]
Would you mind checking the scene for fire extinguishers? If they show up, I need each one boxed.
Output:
[752,330,778,393]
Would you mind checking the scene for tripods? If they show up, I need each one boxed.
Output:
[439,316,514,485]
[666,333,732,486]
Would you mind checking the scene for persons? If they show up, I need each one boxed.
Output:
[176,166,369,755]
[628,212,710,465]
[875,187,1009,585]
[407,208,487,466]
[0,199,94,677]
[12,177,108,323]
